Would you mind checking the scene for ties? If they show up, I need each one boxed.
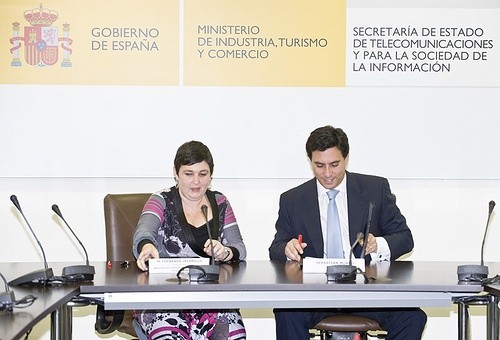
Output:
[326,189,345,259]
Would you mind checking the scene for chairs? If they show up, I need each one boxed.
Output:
[94,193,151,338]
[309,315,387,340]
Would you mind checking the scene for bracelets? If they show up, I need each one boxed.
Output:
[221,251,230,262]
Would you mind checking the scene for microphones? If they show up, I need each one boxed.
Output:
[189,205,220,282]
[7,195,53,286]
[51,204,95,280]
[456,200,496,284]
[325,231,364,282]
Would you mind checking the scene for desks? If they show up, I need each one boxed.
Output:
[0,263,500,340]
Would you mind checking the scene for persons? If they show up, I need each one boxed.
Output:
[269,125,428,340]
[133,141,246,340]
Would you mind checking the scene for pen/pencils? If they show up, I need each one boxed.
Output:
[298,234,302,251]
[120,259,130,265]
